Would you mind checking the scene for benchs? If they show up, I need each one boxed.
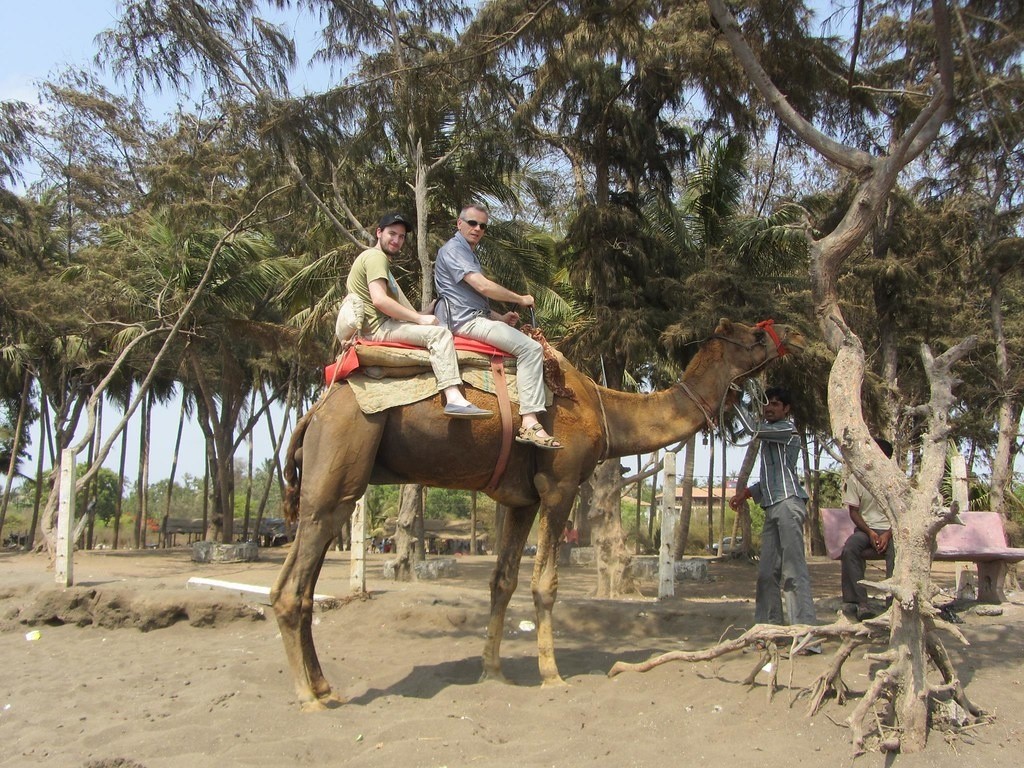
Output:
[155,514,266,549]
[820,506,1024,604]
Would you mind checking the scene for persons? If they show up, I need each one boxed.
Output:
[431,206,566,450]
[727,387,823,658]
[558,520,579,567]
[842,437,896,620]
[370,538,474,553]
[346,214,495,421]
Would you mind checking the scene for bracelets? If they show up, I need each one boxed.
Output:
[743,488,751,500]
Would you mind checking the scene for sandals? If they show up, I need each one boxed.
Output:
[515,423,564,449]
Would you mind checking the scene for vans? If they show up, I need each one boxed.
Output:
[706,536,742,554]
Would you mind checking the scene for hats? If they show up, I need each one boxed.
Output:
[380,214,411,232]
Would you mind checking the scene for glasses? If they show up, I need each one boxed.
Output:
[460,218,487,230]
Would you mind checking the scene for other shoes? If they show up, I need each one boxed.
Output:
[855,603,874,622]
[754,640,787,649]
[798,649,815,656]
[443,403,494,419]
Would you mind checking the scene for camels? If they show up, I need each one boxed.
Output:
[273,316,810,716]
